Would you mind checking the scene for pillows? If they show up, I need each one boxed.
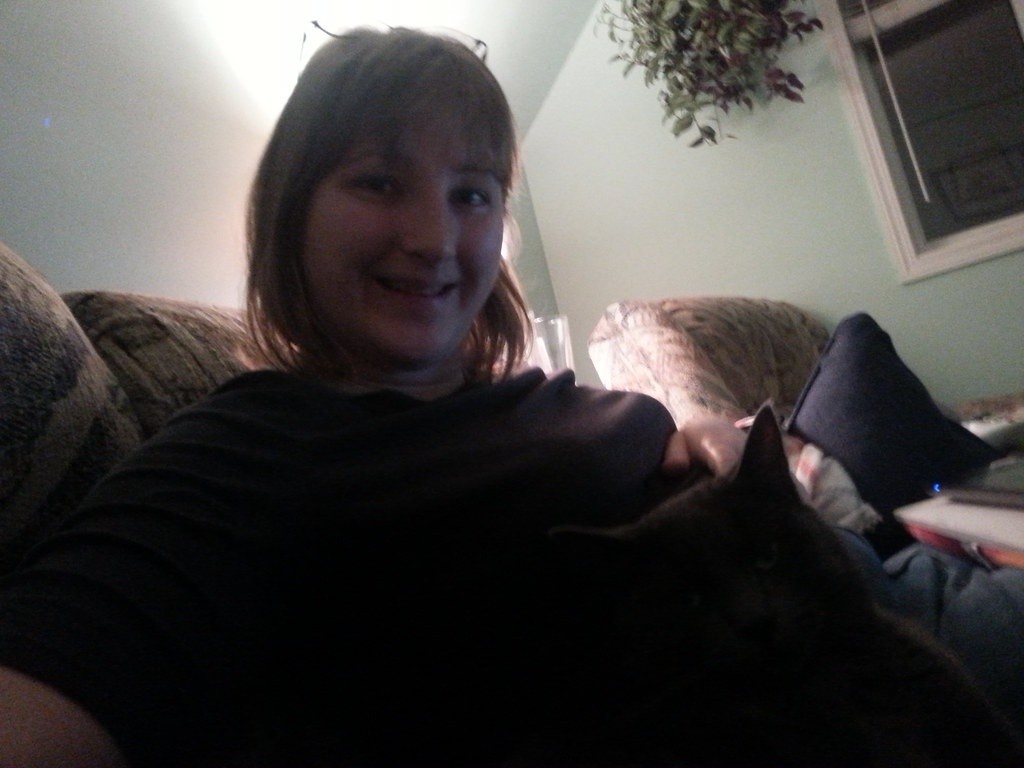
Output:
[779,309,996,560]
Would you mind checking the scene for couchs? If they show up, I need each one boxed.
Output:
[0,243,299,595]
[586,297,1024,536]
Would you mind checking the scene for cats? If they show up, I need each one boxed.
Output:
[544,401,1024,768]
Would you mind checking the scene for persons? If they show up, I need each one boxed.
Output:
[0,27,1023,768]
